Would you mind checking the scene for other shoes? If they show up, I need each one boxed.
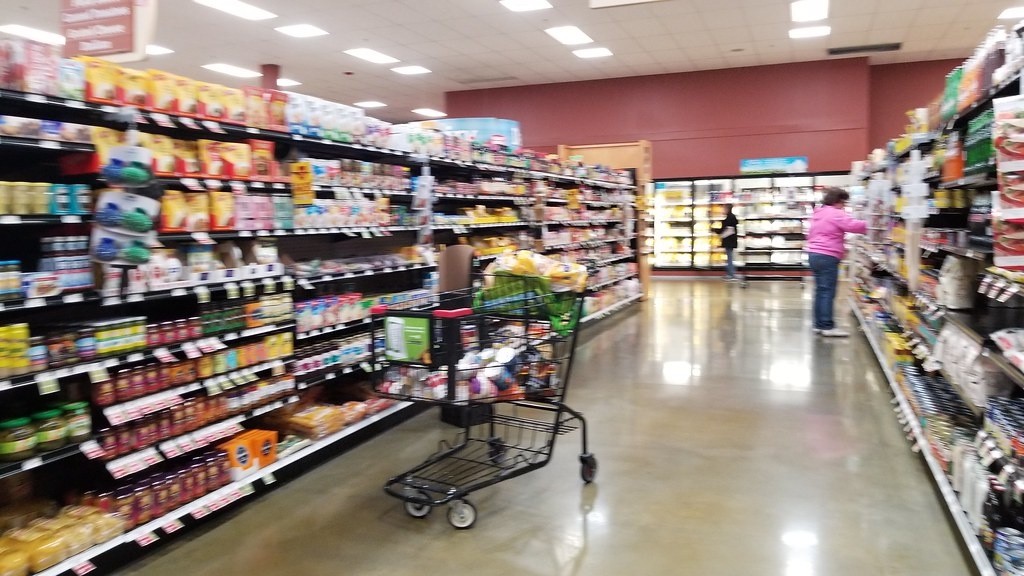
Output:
[721,273,739,281]
[820,327,850,337]
[810,326,822,333]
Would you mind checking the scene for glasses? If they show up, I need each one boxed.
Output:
[839,199,850,204]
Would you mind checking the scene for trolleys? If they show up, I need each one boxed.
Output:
[364,255,597,531]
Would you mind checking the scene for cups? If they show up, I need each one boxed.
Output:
[479,346,521,390]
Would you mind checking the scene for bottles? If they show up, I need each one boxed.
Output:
[979,475,1009,557]
[521,356,557,397]
[925,26,1007,181]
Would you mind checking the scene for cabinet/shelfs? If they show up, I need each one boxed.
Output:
[847,67,1024,576]
[0,89,654,576]
[640,170,869,270]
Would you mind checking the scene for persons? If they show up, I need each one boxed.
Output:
[807,188,867,338]
[709,203,740,282]
